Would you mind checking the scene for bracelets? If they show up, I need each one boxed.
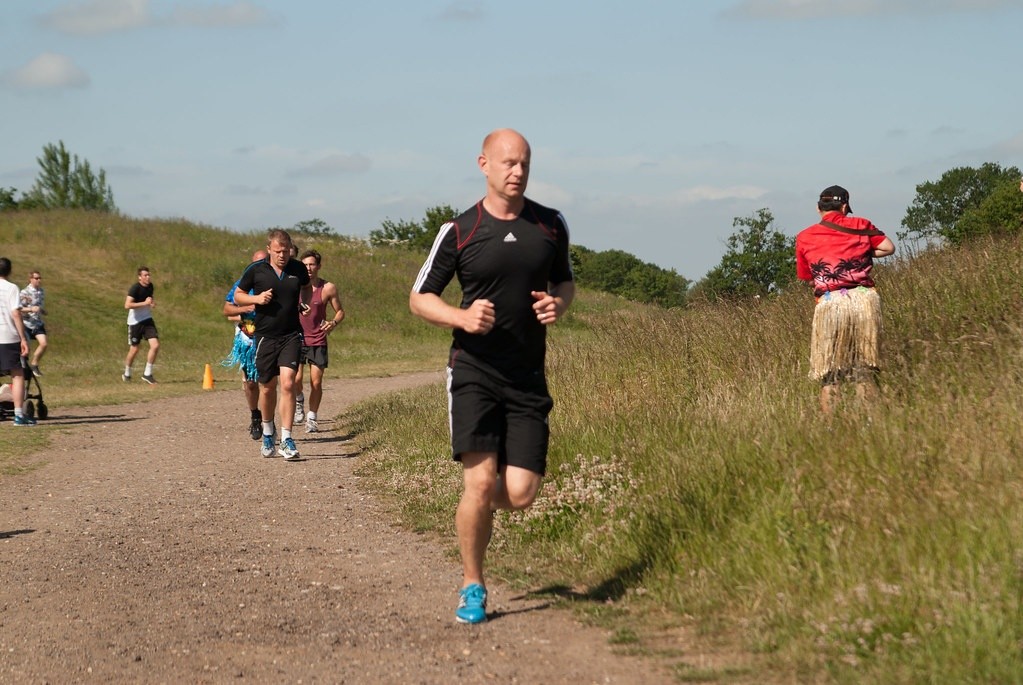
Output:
[330,320,337,327]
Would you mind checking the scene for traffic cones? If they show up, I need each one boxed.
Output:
[202,363,215,391]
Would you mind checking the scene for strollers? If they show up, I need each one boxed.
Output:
[0,356,48,422]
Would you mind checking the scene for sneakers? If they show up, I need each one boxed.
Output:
[306,418,319,434]
[278,438,300,461]
[30,364,42,377]
[122,374,131,382]
[294,398,305,423]
[249,411,263,440]
[261,430,277,458]
[456,585,488,623]
[141,375,157,384]
[14,415,37,426]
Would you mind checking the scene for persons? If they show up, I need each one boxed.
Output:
[796,185,896,413]
[0,257,36,426]
[20,272,48,377]
[236,230,312,459]
[122,267,160,384]
[289,245,299,258]
[409,129,576,624]
[293,251,345,433]
[223,251,277,440]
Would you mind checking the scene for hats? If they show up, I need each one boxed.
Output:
[821,185,854,213]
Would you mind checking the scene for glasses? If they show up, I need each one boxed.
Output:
[33,277,41,280]
